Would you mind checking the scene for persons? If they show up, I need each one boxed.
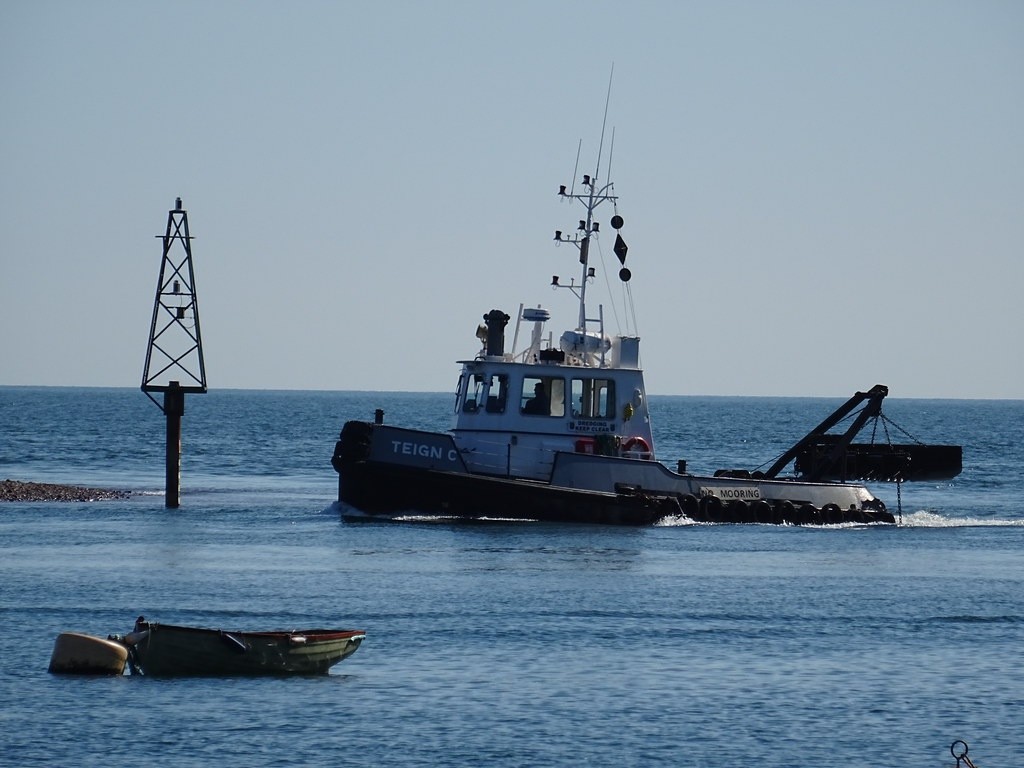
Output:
[525,383,548,408]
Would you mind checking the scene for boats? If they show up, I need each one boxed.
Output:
[329,59,899,527]
[797,443,963,484]
[120,614,367,679]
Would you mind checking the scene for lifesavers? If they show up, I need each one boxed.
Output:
[677,494,700,521]
[751,500,774,524]
[729,499,750,523]
[846,509,865,524]
[799,503,820,525]
[878,512,895,523]
[624,436,651,460]
[821,502,845,525]
[775,499,797,524]
[699,494,723,521]
